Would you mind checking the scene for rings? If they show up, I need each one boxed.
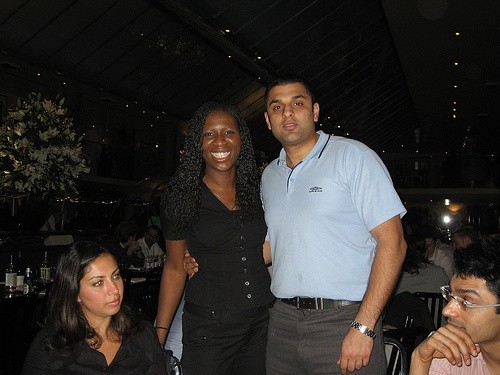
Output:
[185,263,189,271]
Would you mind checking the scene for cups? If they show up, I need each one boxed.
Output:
[147,253,166,270]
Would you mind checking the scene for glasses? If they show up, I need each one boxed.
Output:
[440,285,500,311]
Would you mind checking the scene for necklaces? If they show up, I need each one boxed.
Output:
[141,237,155,260]
[204,177,237,210]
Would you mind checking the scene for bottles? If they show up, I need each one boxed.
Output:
[32,270,40,294]
[5,254,18,289]
[15,250,25,292]
[40,250,51,283]
[23,265,33,297]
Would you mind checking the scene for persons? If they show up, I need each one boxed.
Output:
[186,74,407,374]
[445,229,494,281]
[435,233,454,263]
[408,239,500,375]
[155,100,270,374]
[24,239,170,375]
[416,227,452,280]
[108,221,145,313]
[137,225,166,309]
[384,232,451,330]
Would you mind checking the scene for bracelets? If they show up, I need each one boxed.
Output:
[156,326,169,331]
[135,246,142,252]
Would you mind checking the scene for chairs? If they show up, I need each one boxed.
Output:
[411,291,449,328]
[382,337,408,375]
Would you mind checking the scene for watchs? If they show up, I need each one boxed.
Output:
[350,321,377,339]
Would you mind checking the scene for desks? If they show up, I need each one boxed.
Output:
[0,263,165,353]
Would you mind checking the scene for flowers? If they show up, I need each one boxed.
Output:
[0,95,91,199]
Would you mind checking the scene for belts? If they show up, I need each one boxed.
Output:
[280,298,359,309]
